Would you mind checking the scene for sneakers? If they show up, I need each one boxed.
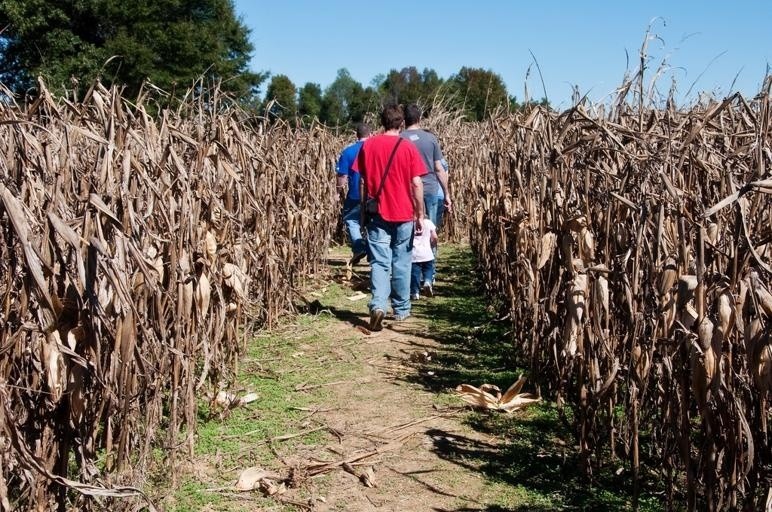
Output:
[348,249,367,267]
[369,279,434,333]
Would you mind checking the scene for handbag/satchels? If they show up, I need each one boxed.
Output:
[359,197,381,230]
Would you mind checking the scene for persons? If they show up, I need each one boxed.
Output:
[336,106,454,329]
